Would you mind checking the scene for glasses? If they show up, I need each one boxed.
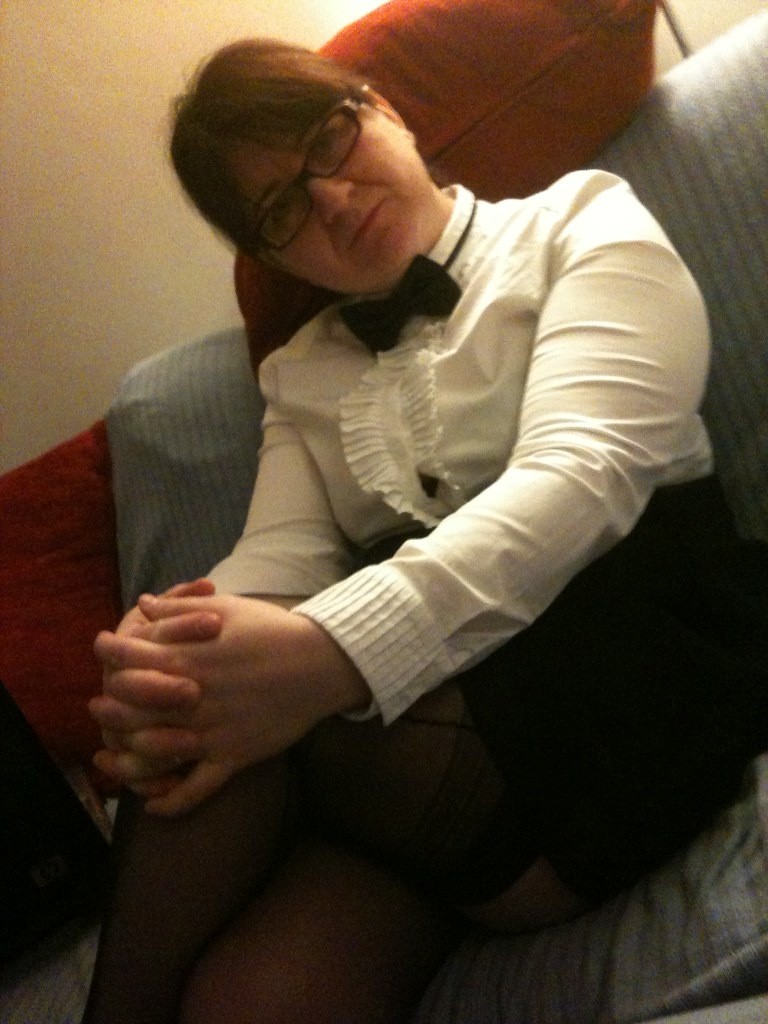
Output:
[246,90,363,260]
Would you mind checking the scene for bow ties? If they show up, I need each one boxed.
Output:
[339,202,478,355]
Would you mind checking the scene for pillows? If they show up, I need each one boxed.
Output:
[232,0,661,340]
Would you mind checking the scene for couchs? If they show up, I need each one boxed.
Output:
[0,9,768,1024]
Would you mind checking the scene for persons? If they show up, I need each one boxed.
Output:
[83,37,767,1024]
